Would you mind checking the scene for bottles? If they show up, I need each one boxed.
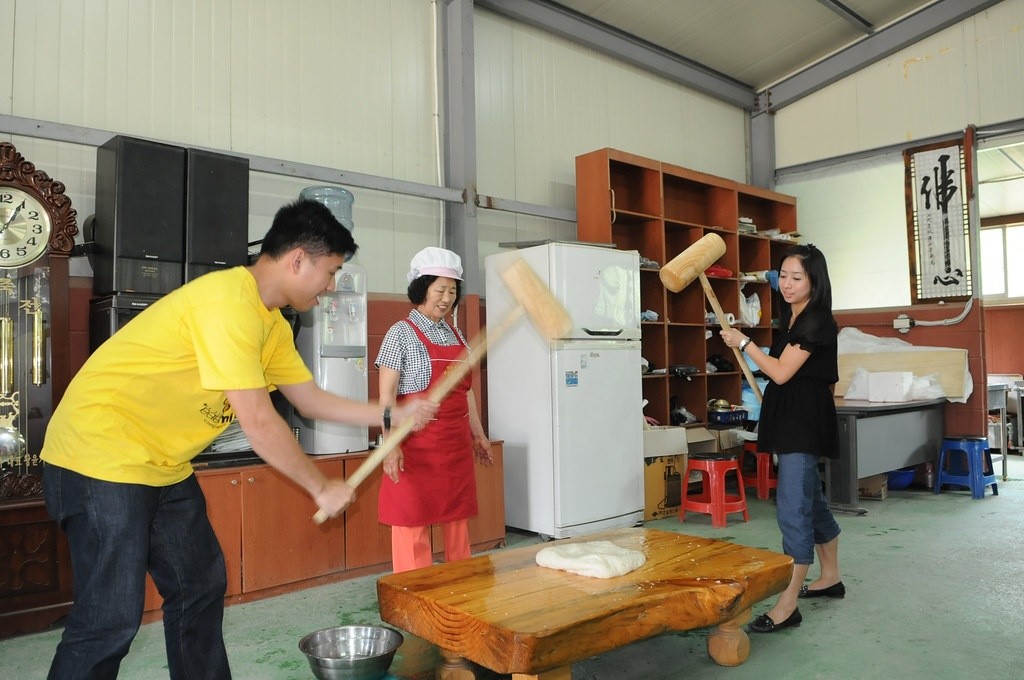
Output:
[298,186,355,264]
[924,464,936,488]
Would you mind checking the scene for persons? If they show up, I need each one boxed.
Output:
[38,196,441,680]
[718,243,846,633]
[375,246,494,577]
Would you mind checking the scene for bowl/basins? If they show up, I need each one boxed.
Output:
[298,624,404,680]
[887,469,917,489]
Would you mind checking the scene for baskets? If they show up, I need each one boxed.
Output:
[709,410,748,426]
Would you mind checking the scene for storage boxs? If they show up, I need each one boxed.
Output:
[856,474,888,501]
[709,427,744,458]
[642,425,688,520]
[685,426,717,483]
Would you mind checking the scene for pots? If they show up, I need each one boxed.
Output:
[669,362,700,381]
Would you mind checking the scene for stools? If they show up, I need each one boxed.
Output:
[933,434,999,500]
[678,452,749,529]
[737,439,778,499]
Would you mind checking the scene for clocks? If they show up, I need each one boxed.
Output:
[0,142,81,506]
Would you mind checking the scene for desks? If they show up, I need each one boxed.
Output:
[986,383,1011,482]
[817,396,949,515]
[376,527,794,680]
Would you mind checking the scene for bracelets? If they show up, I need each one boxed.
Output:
[383,406,391,429]
[739,337,752,352]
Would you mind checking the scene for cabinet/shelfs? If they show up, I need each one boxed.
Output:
[574,147,797,432]
[345,445,505,579]
[139,459,345,626]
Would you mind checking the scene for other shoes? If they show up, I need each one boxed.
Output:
[747,608,802,631]
[798,581,846,599]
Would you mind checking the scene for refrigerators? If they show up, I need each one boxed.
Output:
[484,242,645,540]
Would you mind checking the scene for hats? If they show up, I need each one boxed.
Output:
[408,246,464,281]
